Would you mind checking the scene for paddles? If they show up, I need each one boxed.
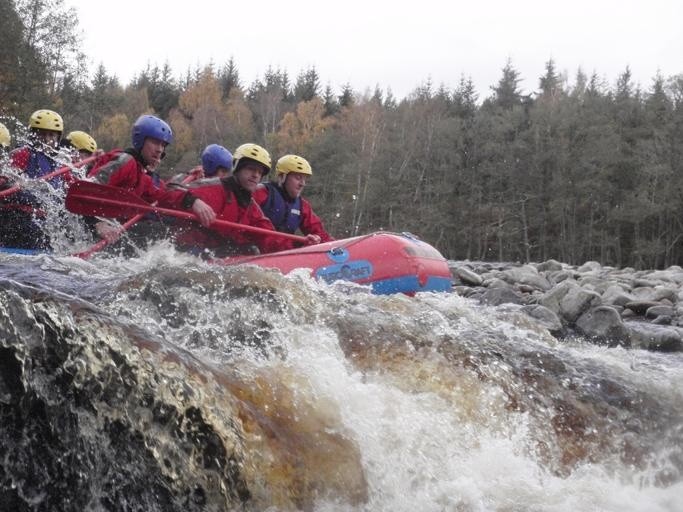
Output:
[66,180,308,243]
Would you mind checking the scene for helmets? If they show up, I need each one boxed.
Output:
[67,131,98,154]
[31,109,64,142]
[232,143,272,176]
[0,121,11,147]
[202,144,233,176]
[275,154,312,184]
[132,115,173,153]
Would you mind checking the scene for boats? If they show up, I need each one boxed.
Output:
[0,227,454,304]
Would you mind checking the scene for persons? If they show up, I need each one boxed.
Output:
[170,143,334,259]
[1,110,172,257]
